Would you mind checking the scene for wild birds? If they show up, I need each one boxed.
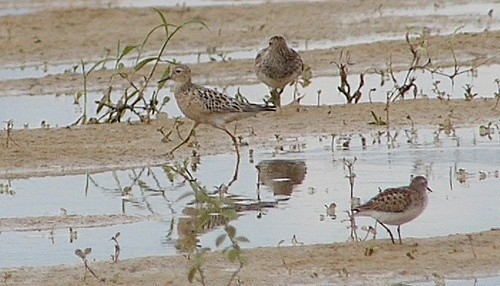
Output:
[163,154,279,253]
[351,175,433,245]
[256,147,307,201]
[157,64,277,154]
[253,35,304,107]
